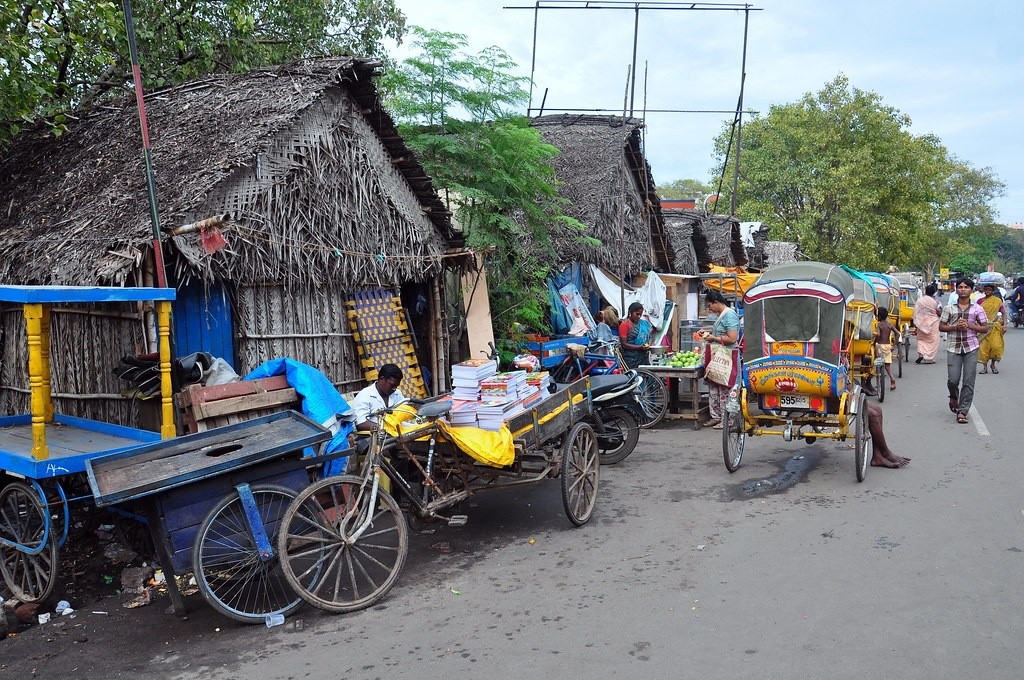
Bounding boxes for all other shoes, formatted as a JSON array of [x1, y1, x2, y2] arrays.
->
[[978, 369, 988, 374], [990, 364, 998, 374], [712, 422, 723, 429], [701, 419, 721, 427]]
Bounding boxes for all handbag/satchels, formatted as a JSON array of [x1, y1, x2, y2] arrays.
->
[[702, 334, 739, 390]]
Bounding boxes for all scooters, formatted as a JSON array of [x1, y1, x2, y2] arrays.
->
[[480, 341, 645, 465]]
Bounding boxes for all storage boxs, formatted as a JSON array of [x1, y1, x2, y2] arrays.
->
[[495, 333, 590, 371]]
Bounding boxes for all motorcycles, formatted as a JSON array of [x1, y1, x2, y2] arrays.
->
[[1007, 297, 1024, 328]]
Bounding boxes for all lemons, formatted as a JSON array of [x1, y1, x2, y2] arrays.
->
[[666, 351, 700, 367]]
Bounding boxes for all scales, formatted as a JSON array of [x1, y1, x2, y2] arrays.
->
[[648, 346, 669, 364]]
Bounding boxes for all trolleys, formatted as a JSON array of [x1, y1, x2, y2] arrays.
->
[[145, 447, 361, 624]]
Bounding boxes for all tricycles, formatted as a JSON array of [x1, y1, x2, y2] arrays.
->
[[844, 264, 919, 403], [277, 379, 600, 614], [515, 334, 668, 429], [722, 261, 872, 483]]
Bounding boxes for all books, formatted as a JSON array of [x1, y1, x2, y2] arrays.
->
[[437, 359, 550, 431]]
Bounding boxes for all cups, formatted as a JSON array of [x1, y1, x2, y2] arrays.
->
[[266, 613, 285, 629]]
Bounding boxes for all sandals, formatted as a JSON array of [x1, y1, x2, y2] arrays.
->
[[957, 410, 968, 423], [949, 397, 959, 413]]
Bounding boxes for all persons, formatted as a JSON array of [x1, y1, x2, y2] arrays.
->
[[350, 363, 420, 510], [696, 291, 741, 429], [836, 277, 1024, 468], [618, 302, 679, 422]]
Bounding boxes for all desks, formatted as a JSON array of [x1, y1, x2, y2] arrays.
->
[[638, 364, 711, 431]]
